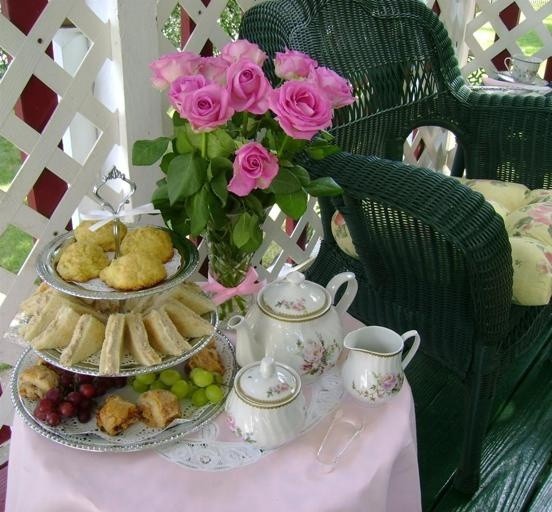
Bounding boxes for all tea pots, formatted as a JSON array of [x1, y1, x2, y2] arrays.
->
[[341, 326, 421, 404], [228, 271, 357, 378]]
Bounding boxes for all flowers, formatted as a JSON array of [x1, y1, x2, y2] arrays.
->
[[133, 40, 356, 316]]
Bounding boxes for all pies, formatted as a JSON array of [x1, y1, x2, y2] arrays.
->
[[17, 366, 59, 402], [96, 394, 141, 437], [136, 388, 182, 428]]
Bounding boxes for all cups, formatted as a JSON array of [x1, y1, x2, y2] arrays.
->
[[505, 55, 543, 85]]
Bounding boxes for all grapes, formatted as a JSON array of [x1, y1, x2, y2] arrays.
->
[[132, 368, 224, 406], [33, 371, 126, 426]]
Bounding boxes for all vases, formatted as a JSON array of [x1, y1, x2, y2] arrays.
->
[[205, 210, 259, 289]]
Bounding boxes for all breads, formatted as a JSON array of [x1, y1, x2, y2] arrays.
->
[[57, 219, 173, 290]]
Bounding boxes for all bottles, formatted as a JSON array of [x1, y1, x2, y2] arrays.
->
[[223, 358, 307, 450]]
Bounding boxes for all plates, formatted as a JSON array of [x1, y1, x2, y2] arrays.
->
[[497, 70, 549, 87]]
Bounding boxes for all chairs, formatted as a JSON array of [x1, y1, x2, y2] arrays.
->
[[239, 0, 552, 494]]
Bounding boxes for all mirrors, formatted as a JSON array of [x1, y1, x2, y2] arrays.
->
[[395, 118, 469, 180]]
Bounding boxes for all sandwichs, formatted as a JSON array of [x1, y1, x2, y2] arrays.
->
[[19, 281, 216, 376]]
